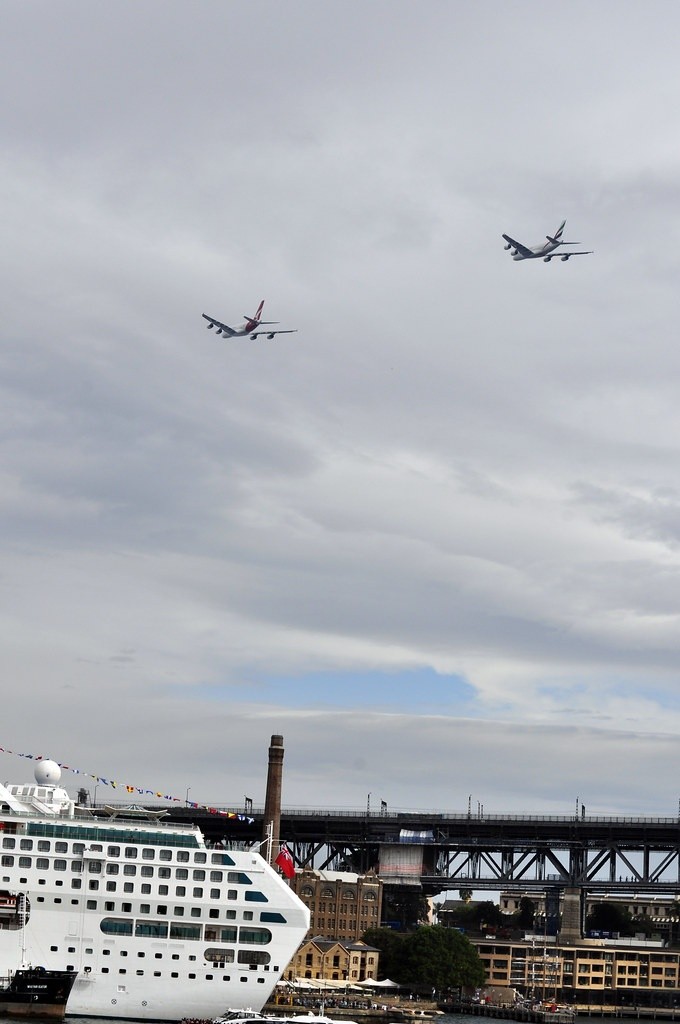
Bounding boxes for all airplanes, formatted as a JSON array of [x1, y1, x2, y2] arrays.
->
[[202, 300, 298, 340], [502, 219, 594, 262]]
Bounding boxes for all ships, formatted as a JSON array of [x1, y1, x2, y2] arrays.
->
[[0, 759, 311, 1024]]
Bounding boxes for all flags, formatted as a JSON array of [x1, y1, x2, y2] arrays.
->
[[0, 748, 255, 824], [275, 844, 297, 880]]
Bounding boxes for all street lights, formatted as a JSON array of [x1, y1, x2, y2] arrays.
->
[[367, 792, 372, 817], [468, 794, 472, 819], [186, 788, 191, 807], [94, 785, 100, 808], [575, 795, 579, 823]]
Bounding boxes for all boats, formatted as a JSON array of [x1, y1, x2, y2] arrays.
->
[[399, 1008, 445, 1020], [181, 992, 357, 1024], [1, 966, 80, 1023]]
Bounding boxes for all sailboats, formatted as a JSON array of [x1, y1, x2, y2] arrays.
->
[[514, 917, 576, 1013]]
